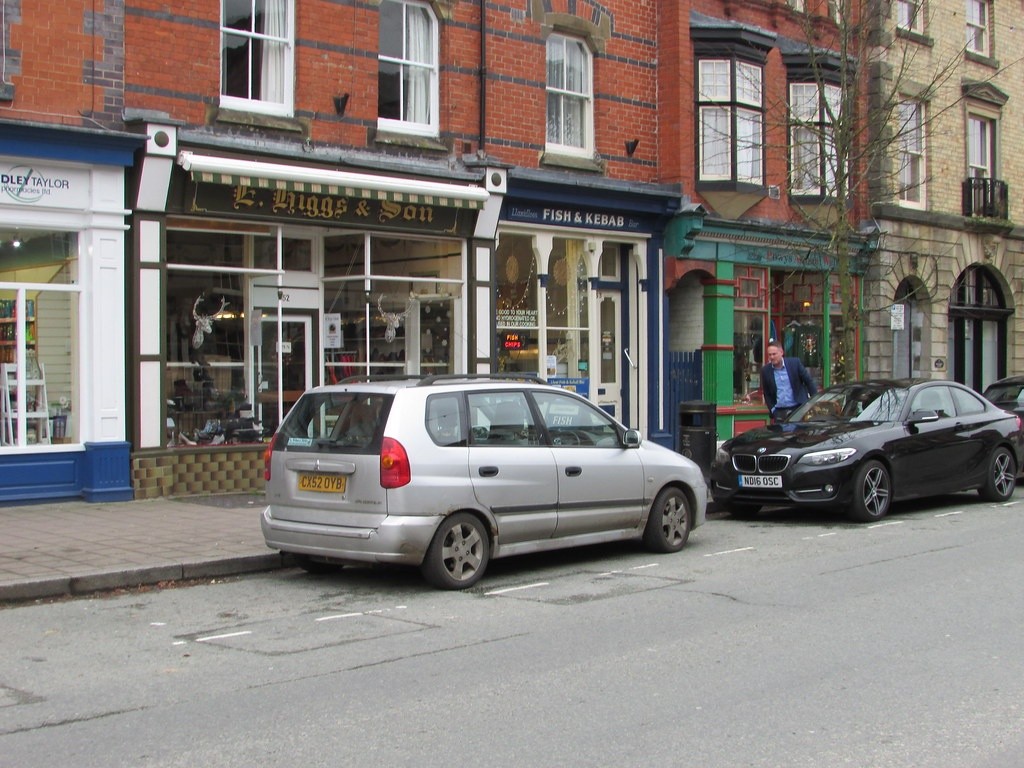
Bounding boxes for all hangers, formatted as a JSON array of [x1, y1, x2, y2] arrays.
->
[[781, 312, 815, 331]]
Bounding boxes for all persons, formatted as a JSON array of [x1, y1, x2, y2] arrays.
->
[[761, 341, 817, 423]]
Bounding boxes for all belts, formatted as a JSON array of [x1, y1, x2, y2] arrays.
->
[[773, 405, 802, 411]]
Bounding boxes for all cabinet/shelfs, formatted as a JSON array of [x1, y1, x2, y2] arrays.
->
[[0, 299, 38, 375], [342, 296, 457, 379], [166, 356, 231, 435]]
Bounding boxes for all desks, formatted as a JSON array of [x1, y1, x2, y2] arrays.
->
[[256, 389, 305, 402]]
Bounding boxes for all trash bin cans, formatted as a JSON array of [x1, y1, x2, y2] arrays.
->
[[677, 399, 718, 490]]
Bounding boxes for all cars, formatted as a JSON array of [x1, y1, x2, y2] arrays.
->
[[0, 387, 71, 446], [260, 373, 708, 590], [983, 375, 1023, 423], [710, 377, 1024, 523]]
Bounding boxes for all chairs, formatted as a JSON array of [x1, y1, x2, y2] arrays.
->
[[486, 399, 528, 447], [921, 391, 946, 416]]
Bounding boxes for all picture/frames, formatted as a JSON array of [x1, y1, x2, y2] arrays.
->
[[410, 271, 440, 296]]
